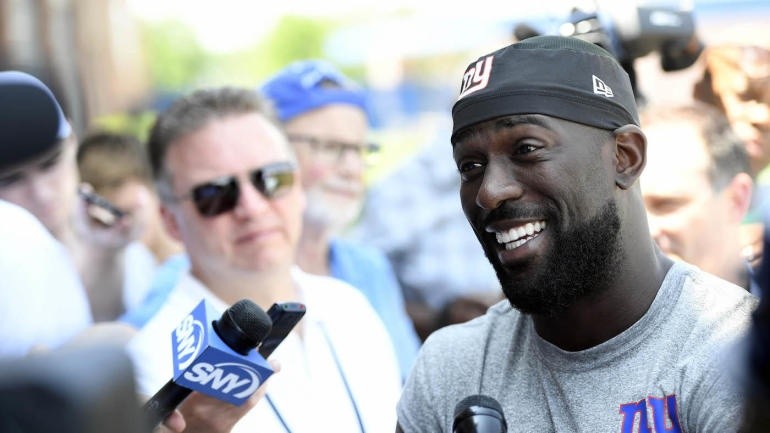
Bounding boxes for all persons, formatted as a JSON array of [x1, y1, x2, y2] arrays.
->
[[0, 71, 188, 363], [363, 132, 506, 330], [394, 34, 761, 433], [129, 87, 402, 433], [265, 63, 421, 391], [639, 22, 770, 296]]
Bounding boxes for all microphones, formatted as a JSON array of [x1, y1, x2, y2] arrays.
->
[[141, 300, 271, 433], [450, 393, 508, 433]]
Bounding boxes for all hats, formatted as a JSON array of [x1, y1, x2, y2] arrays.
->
[[450, 35, 639, 132], [262, 59, 384, 128], [0, 71, 73, 164]]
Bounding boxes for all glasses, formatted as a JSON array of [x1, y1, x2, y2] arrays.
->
[[169, 160, 296, 218], [287, 132, 384, 164]]
